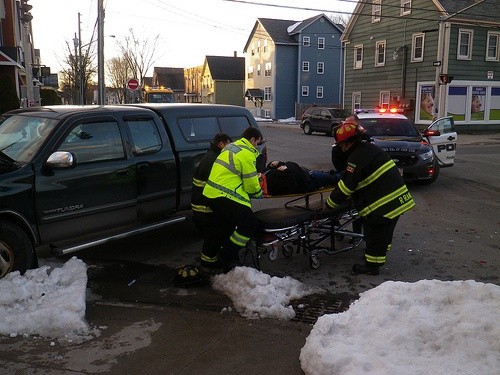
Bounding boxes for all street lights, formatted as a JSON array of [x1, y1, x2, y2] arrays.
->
[[78, 34, 115, 104]]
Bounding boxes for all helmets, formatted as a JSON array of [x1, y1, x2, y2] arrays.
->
[[174, 262, 207, 288], [332, 123, 365, 143]]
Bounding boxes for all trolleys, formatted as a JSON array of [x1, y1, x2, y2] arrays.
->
[[237, 188, 365, 270]]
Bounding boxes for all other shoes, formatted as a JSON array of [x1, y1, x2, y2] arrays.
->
[[352, 259, 381, 275], [199, 257, 224, 268]]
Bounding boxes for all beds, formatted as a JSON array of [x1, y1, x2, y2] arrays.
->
[[243, 191, 365, 270]]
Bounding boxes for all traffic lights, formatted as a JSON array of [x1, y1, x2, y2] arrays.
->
[[439, 73, 455, 83]]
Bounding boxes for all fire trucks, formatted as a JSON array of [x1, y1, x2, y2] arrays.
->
[[143, 85, 175, 104]]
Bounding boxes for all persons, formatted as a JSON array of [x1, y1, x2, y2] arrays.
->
[[257, 160, 346, 196], [200, 127, 265, 268], [323, 114, 417, 275], [190, 132, 240, 263]]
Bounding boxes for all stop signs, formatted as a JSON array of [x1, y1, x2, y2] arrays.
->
[[127, 79, 138, 91]]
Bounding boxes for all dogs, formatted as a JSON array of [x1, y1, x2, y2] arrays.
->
[[471, 95, 482, 113], [422, 93, 434, 116]]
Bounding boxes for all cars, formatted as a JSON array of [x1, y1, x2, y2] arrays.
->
[[331, 108, 458, 187]]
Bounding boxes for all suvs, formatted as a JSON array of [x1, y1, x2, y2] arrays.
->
[[0, 102, 269, 280], [300, 106, 351, 137]]
